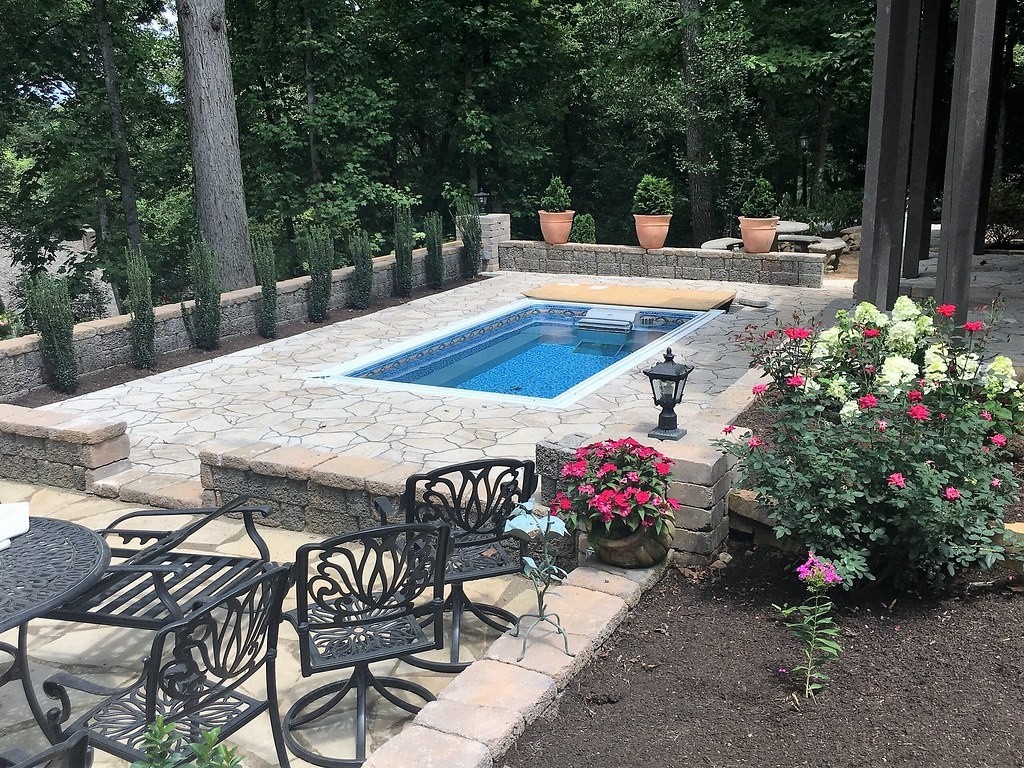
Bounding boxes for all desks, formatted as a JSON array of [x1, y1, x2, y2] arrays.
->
[[0, 517, 111, 768]]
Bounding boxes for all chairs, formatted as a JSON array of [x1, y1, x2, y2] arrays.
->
[[282, 525, 451, 768], [374, 459, 538, 672], [43, 563, 290, 768], [39, 493, 273, 662]]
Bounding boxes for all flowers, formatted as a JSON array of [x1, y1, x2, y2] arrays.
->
[[550, 438, 680, 538]]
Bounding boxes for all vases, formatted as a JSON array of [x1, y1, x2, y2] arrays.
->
[[586, 503, 676, 568]]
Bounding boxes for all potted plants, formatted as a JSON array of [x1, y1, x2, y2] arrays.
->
[[538, 175, 576, 244], [738, 178, 780, 253], [632, 173, 675, 248]]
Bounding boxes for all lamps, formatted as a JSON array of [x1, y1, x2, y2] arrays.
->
[[643, 347, 694, 440], [475, 188, 490, 216]]
